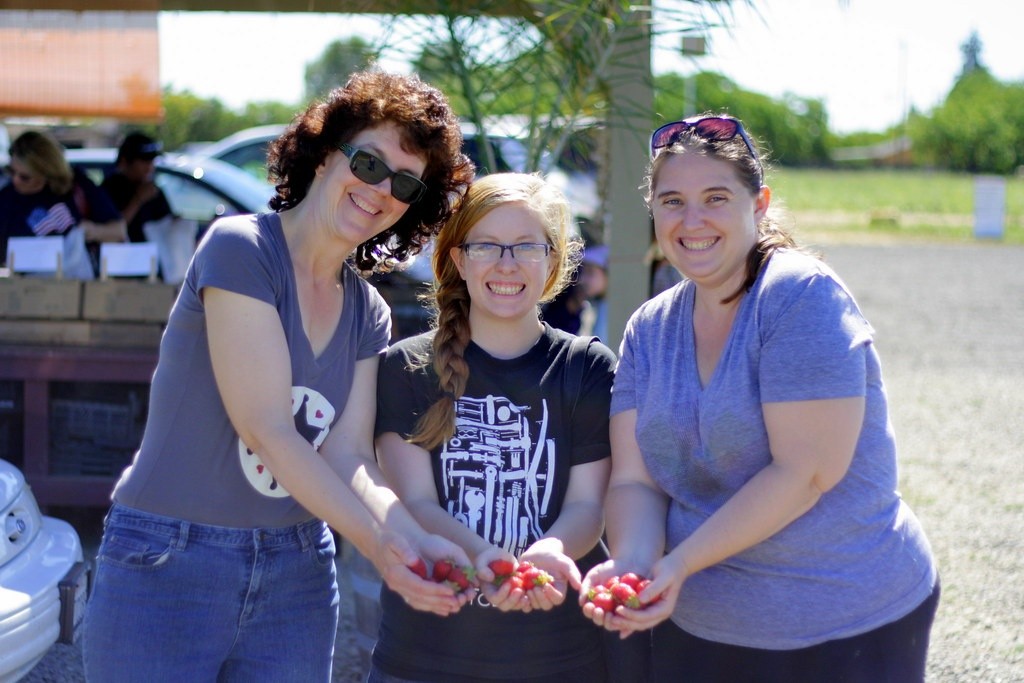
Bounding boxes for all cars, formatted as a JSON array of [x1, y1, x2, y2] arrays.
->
[[1, 150, 442, 348], [486, 109, 606, 244], [0, 458, 94, 683]]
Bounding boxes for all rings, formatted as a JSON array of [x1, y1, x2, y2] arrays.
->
[[491, 601, 499, 609], [404, 596, 414, 608]]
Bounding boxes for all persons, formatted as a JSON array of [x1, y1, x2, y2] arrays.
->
[[0, 130, 129, 470], [576, 109, 941, 682], [79, 69, 475, 683], [99, 128, 186, 420], [367, 169, 619, 683]]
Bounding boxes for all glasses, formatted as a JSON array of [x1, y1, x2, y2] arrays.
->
[[5, 165, 45, 182], [458, 239, 556, 262], [339, 146, 428, 206], [649, 117, 760, 167]]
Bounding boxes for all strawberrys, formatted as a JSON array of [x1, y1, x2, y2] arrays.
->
[[411, 558, 552, 596], [586, 571, 658, 614]]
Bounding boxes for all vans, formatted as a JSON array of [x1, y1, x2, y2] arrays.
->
[[198, 122, 570, 267]]
